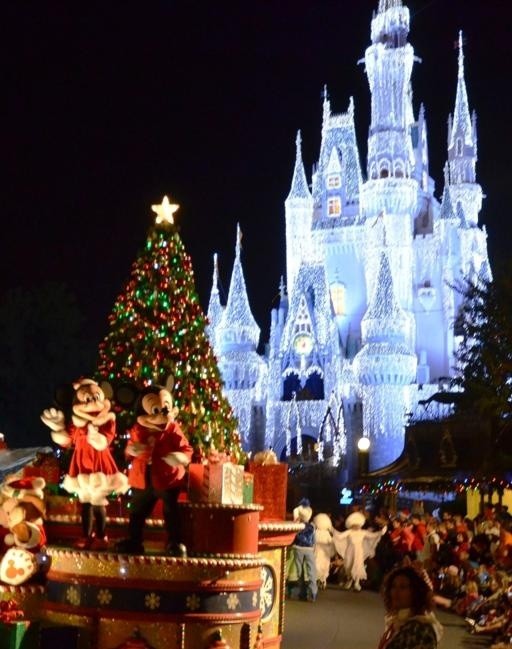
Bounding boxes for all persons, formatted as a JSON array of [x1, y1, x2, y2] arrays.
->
[[327, 498, 512, 649], [285, 497, 387, 602]]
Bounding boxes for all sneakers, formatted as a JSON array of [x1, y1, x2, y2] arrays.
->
[[353, 584, 362, 591], [343, 581, 353, 590]]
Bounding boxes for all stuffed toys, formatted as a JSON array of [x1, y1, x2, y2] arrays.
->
[[40, 374, 131, 551], [113, 374, 194, 556]]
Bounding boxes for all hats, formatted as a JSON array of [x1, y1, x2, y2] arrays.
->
[[293, 505, 313, 524], [313, 513, 332, 529], [346, 512, 367, 528]]
